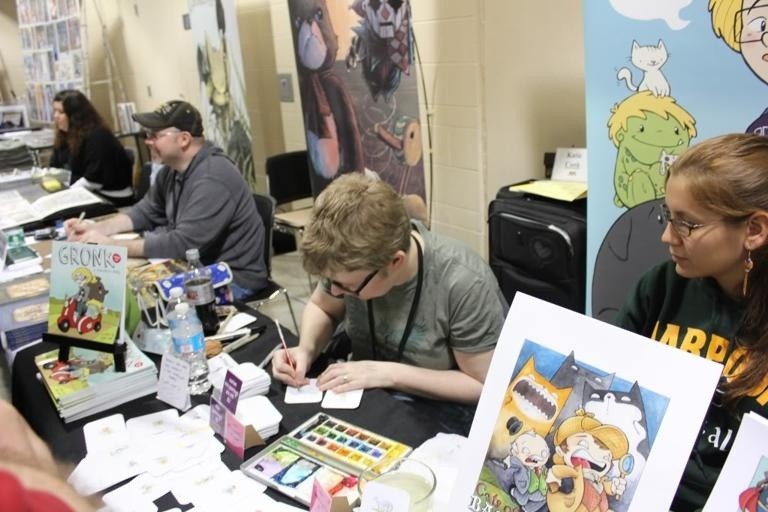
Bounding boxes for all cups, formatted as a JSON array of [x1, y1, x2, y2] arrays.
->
[[355, 457, 439, 512]]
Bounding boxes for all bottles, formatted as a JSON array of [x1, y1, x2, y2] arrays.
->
[[165, 287, 216, 397], [181, 246, 220, 336]]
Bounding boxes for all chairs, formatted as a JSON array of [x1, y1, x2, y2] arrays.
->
[[265, 150, 315, 253], [124, 145, 138, 183], [246, 193, 301, 339]]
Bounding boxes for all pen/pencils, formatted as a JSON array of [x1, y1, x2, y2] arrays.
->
[[258, 343, 284, 369], [204, 324, 268, 353], [66, 212, 86, 243]]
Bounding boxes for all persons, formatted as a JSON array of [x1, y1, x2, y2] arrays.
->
[[271, 170, 508, 438], [0, 400, 94, 512], [47, 90, 136, 207], [614, 133, 768, 512], [62, 101, 268, 300]]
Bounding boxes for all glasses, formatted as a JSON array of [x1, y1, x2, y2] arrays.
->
[[141, 127, 177, 139], [660, 203, 724, 237], [331, 269, 377, 296]]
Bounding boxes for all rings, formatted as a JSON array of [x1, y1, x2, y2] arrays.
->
[[344, 374, 348, 382]]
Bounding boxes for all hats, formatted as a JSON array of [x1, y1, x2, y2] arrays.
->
[[133, 101, 203, 135]]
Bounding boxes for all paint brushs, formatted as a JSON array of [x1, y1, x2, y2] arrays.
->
[[274, 319, 300, 392]]
